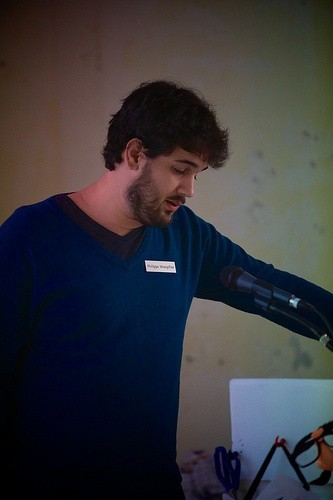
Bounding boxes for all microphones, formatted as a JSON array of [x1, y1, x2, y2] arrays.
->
[[220, 264, 313, 323]]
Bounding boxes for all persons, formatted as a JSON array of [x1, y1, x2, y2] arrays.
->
[[0, 79, 332, 499]]
